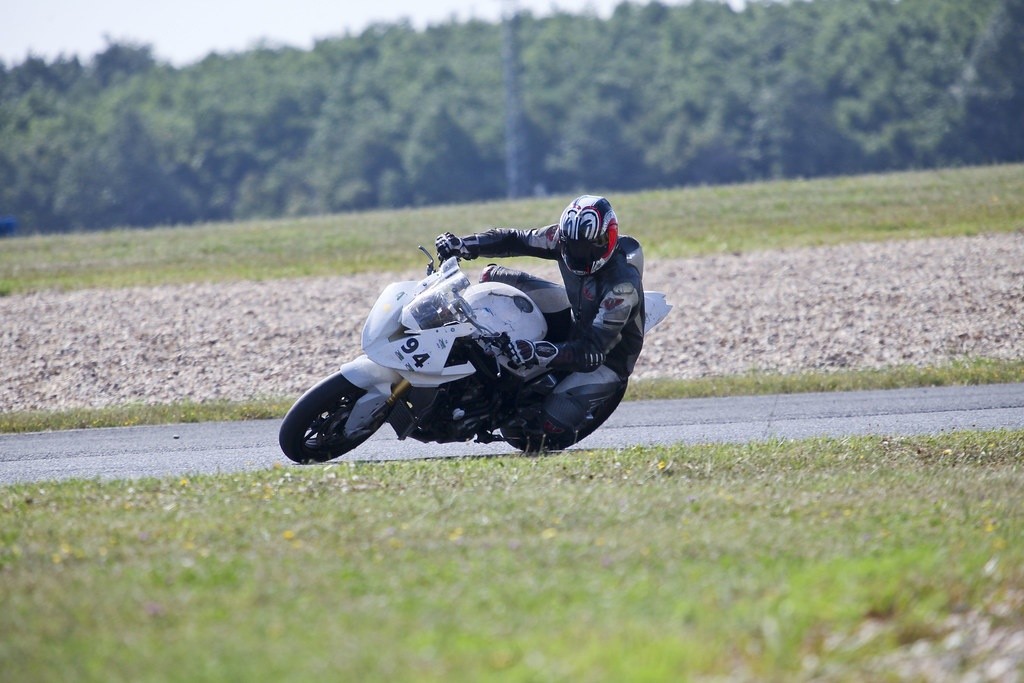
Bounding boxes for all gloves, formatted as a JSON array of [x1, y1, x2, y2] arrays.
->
[[504, 339, 557, 369], [434, 232, 479, 261]]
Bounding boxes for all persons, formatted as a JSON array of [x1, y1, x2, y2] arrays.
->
[[436, 195, 646, 439]]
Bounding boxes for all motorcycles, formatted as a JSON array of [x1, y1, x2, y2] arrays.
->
[[277, 230, 673, 465]]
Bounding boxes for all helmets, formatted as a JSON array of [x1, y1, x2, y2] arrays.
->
[[558, 195, 621, 277]]
